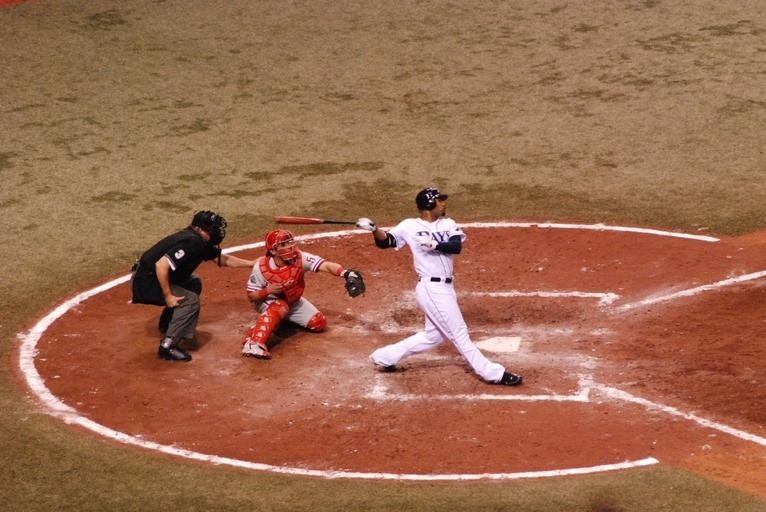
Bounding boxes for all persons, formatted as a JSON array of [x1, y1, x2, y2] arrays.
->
[[131, 210, 266, 363], [352, 187, 526, 388], [241, 230, 365, 358]]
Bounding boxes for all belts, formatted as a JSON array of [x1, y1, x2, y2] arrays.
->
[[430, 277, 453, 284]]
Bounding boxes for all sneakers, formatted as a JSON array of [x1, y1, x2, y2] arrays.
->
[[242, 337, 272, 358], [494, 372, 521, 385], [370, 355, 394, 371]]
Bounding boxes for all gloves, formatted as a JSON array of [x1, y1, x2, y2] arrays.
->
[[340, 269, 359, 278], [356, 218, 376, 232], [418, 237, 439, 252]]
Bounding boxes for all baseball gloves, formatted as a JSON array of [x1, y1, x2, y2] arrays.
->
[[345, 269, 365, 296]]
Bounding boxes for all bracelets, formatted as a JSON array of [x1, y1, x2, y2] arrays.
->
[[259, 286, 268, 300]]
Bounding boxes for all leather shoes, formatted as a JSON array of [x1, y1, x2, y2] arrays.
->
[[159, 345, 191, 361]]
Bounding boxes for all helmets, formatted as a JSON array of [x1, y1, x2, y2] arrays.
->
[[265, 229, 298, 261], [415, 187, 448, 210], [192, 210, 226, 245]]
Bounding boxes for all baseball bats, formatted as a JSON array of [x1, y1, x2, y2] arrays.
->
[[275, 216, 375, 227]]
